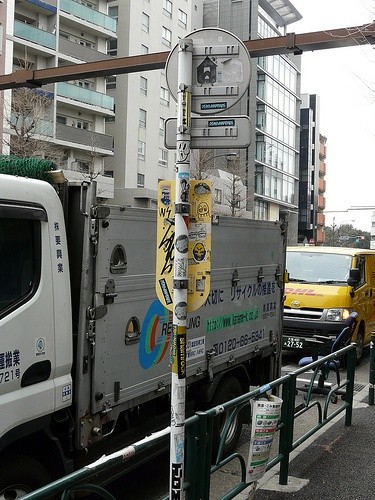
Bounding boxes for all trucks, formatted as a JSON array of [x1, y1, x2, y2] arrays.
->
[[0, 173, 288, 500]]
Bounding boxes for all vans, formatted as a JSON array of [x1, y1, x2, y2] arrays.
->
[[282, 246, 375, 367]]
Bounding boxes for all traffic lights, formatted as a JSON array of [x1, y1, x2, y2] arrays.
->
[[355, 235, 364, 240]]
